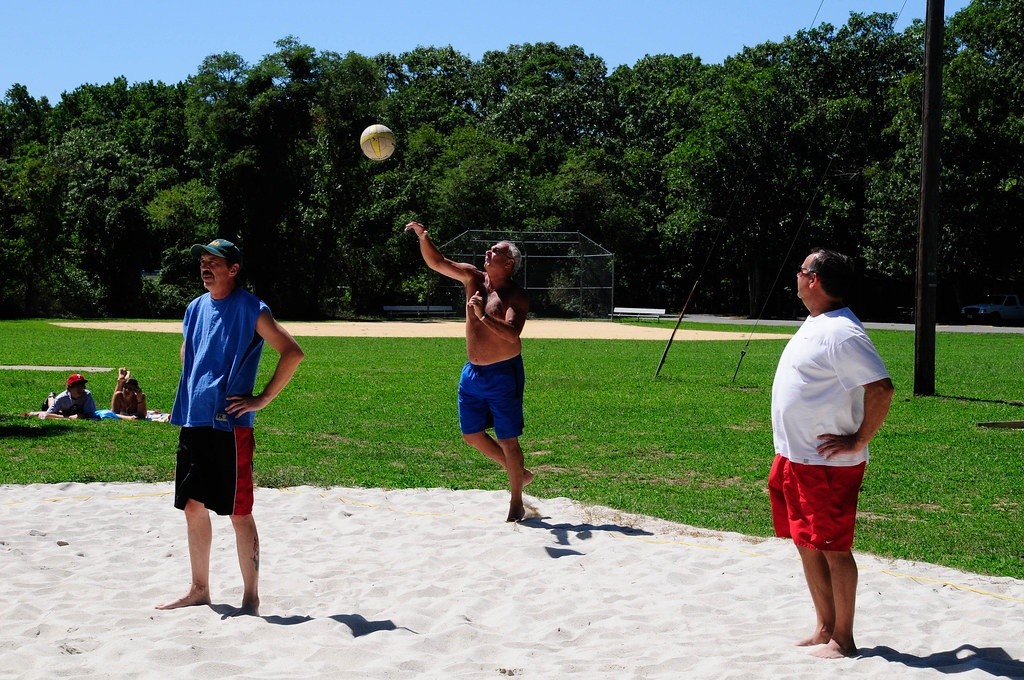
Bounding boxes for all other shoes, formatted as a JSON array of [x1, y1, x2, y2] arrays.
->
[[42, 392, 54, 411]]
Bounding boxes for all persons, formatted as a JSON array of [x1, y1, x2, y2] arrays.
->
[[767, 251, 894, 660], [42, 374, 97, 419], [111, 367, 147, 421], [154, 238, 304, 616], [405, 221, 534, 522]]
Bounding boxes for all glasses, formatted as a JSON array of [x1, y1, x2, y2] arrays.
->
[[797, 265, 818, 276]]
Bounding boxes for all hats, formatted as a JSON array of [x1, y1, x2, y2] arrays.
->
[[191, 239, 239, 260], [123, 379, 138, 390], [67, 374, 87, 387]]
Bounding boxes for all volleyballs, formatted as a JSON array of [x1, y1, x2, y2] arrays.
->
[[360, 124, 396, 161]]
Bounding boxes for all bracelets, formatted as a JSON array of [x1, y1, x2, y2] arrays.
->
[[137, 402, 142, 404], [480, 313, 488, 321]]
